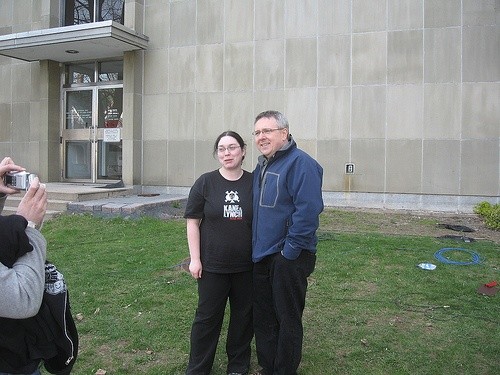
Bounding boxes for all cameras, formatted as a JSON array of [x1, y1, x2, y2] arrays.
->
[[4, 171, 36, 190]]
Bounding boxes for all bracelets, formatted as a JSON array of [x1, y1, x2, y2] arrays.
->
[[26, 221, 42, 232]]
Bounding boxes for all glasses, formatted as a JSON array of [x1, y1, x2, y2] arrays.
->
[[218, 145, 241, 152], [252, 127, 283, 136]]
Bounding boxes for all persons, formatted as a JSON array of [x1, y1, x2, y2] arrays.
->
[[0, 156, 48, 375], [183, 131, 255, 375], [253, 110, 324, 375]]
